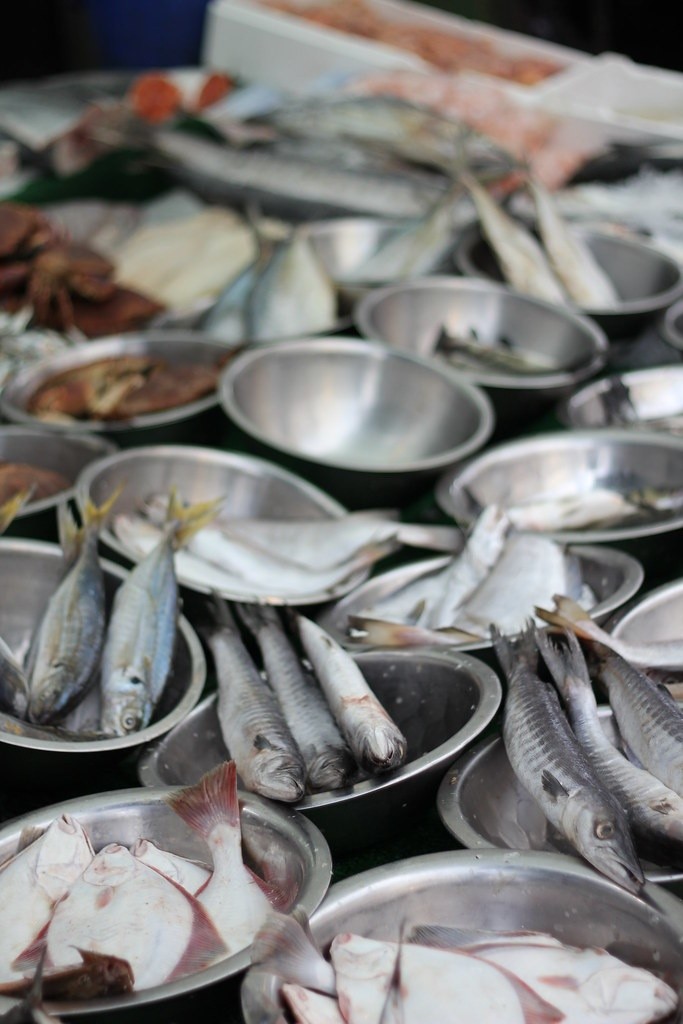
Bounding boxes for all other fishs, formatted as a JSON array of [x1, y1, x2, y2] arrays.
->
[[0, 98, 683, 1024]]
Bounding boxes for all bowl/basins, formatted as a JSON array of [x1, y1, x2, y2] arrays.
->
[[0, 211, 683, 1024]]
